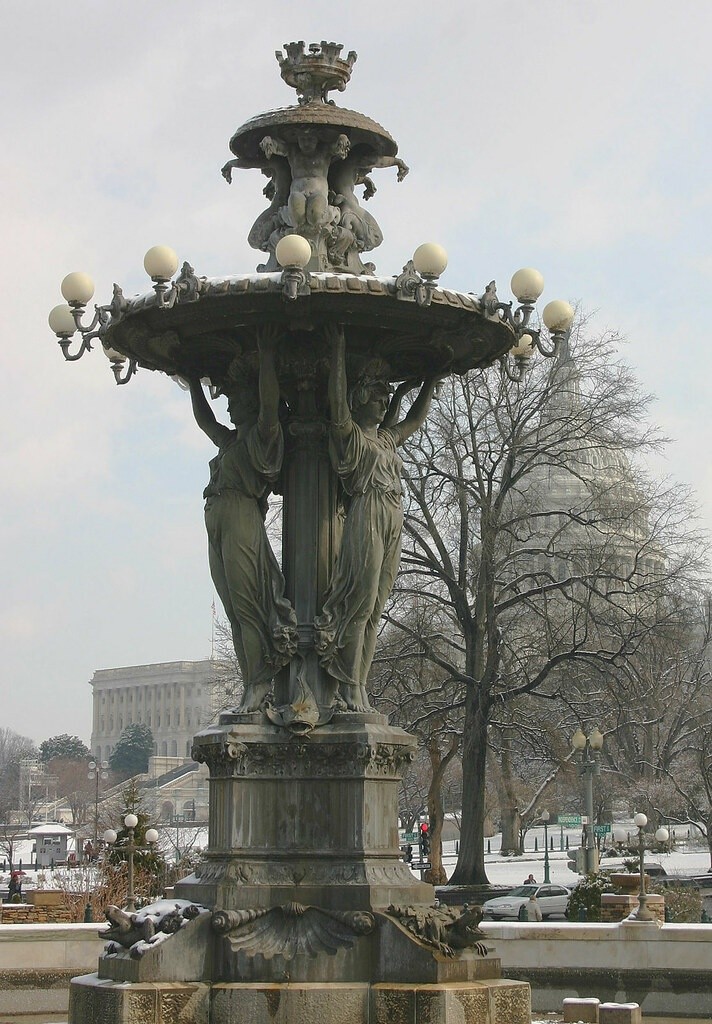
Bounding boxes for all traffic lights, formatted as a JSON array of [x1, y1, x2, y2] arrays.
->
[[404, 846, 412, 863], [566, 848, 586, 873], [420, 823, 430, 855]]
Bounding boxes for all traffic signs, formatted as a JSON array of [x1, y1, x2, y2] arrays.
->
[[411, 863, 432, 870], [591, 825, 611, 837], [557, 815, 581, 828]]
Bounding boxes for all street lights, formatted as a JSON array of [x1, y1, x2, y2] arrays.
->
[[87, 758, 109, 851], [542, 808, 552, 883], [103, 815, 160, 912], [571, 726, 603, 881], [613, 812, 670, 921]]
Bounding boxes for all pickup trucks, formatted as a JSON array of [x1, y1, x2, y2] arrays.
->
[[596, 862, 712, 896]]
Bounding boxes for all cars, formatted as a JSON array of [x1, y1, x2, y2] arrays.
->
[[480, 882, 584, 920]]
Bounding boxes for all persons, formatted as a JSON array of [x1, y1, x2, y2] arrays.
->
[[321, 323, 455, 706], [5, 875, 22, 901], [210, 132, 412, 252], [524, 894, 543, 922], [523, 874, 536, 885], [148, 325, 303, 715]]
